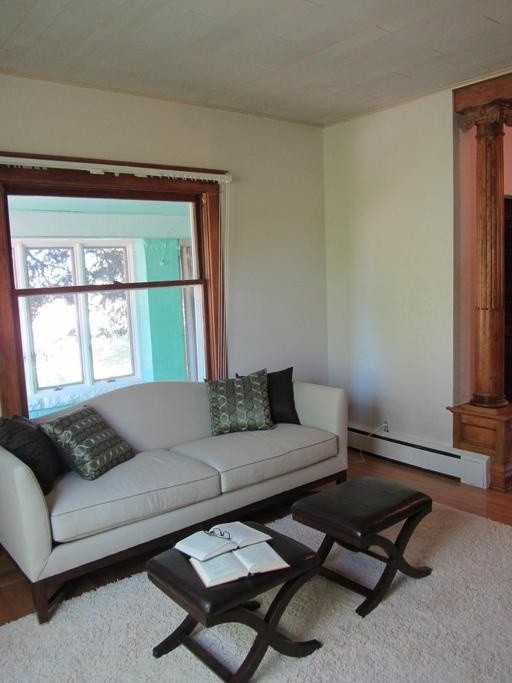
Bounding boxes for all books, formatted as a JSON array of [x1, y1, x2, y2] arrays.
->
[[187, 541, 292, 588], [173, 520, 275, 560]]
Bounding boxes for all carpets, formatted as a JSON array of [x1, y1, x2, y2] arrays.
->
[[0, 501, 511, 683]]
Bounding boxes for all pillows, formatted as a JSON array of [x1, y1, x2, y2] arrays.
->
[[203, 365, 302, 437], [1, 403, 138, 496]]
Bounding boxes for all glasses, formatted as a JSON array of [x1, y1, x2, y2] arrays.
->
[[203, 528, 230, 540]]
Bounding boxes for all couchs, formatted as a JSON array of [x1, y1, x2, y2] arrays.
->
[[1, 379, 349, 625]]
